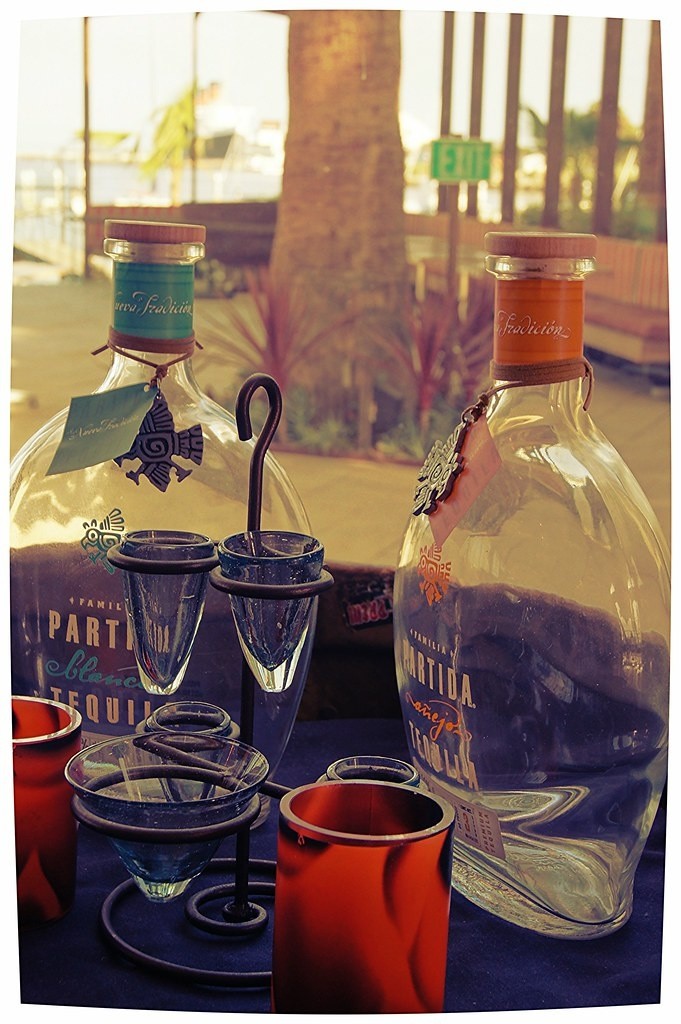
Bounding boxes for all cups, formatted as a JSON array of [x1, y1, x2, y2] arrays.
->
[[269, 778, 458, 1014], [11, 695, 84, 934]]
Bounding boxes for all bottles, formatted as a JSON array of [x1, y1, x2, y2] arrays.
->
[[11, 220, 321, 836], [390, 231, 670, 943]]
